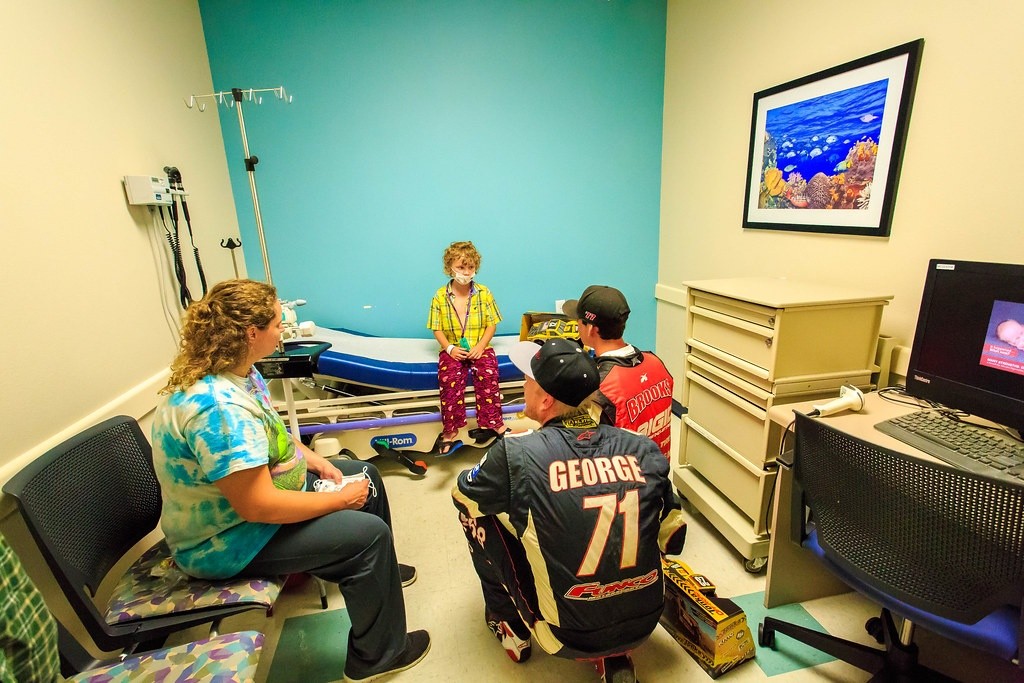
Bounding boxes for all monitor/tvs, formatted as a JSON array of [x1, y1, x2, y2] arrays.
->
[[906, 259, 1024, 444]]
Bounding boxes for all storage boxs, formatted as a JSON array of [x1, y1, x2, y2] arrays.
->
[[660, 555, 756, 680]]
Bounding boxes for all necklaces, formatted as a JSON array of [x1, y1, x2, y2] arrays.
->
[[445, 283, 473, 353]]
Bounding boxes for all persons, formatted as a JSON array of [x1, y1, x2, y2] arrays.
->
[[0, 531, 61, 683], [151, 279, 432, 683], [428, 241, 516, 461], [453, 337, 674, 683], [562, 284, 673, 472], [996, 318, 1024, 350]]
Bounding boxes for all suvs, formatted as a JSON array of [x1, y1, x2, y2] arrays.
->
[[527, 319, 580, 346]]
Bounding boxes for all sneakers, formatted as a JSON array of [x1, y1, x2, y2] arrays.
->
[[398, 564, 416, 587], [597, 653, 639, 683], [343, 630, 431, 683], [485, 605, 532, 662]]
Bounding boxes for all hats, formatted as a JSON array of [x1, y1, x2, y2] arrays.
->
[[562, 285, 631, 326], [508, 338, 599, 408]]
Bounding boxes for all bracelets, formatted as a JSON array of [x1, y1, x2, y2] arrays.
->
[[447, 345, 455, 355]]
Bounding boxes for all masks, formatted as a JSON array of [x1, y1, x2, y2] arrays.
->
[[450, 266, 474, 285], [313, 466, 377, 497]]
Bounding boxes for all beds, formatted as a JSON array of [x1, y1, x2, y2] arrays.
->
[[265, 307, 542, 474]]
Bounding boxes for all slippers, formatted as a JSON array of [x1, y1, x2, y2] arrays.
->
[[434, 439, 463, 457]]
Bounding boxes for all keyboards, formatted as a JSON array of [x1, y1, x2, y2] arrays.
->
[[873, 409, 1024, 485]]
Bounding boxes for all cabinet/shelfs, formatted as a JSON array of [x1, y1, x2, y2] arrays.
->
[[671, 286, 895, 571]]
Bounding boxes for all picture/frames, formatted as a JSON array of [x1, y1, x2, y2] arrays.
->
[[741, 37, 926, 238]]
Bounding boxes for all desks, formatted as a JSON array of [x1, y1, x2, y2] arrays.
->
[[762, 386, 1024, 646]]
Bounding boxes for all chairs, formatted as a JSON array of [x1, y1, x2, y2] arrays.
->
[[757, 408, 1024, 683]]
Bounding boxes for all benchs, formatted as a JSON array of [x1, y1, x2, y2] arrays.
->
[[0, 415, 328, 683]]
